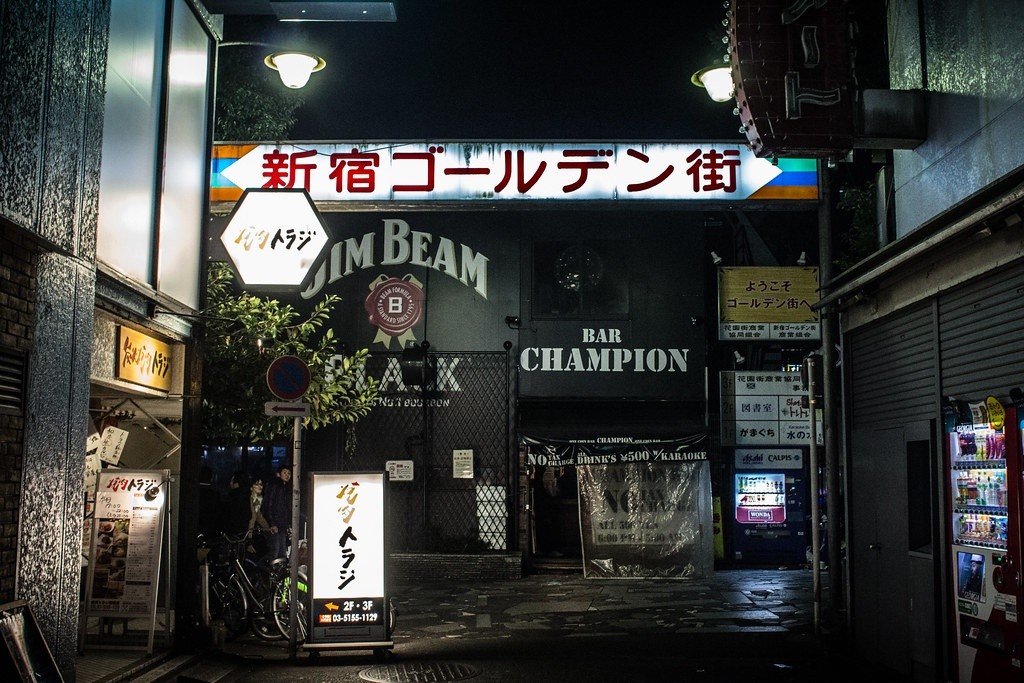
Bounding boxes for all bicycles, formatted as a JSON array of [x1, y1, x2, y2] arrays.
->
[[194, 527, 396, 650]]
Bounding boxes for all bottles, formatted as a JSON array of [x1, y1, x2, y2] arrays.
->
[[957, 429, 1006, 463], [779, 481, 783, 491], [757, 495, 761, 505], [761, 494, 766, 504], [774, 481, 779, 490], [738, 476, 766, 492], [766, 481, 770, 491], [956, 470, 1007, 507], [770, 481, 773, 491], [959, 514, 1007, 542], [775, 494, 783, 504]]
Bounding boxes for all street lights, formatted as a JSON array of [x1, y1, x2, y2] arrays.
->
[[693, 64, 842, 587]]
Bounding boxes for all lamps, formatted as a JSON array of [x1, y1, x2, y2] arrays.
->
[[692, 53, 735, 102], [218, 40, 326, 88]]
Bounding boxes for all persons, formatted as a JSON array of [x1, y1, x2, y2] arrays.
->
[[197, 465, 292, 579]]
